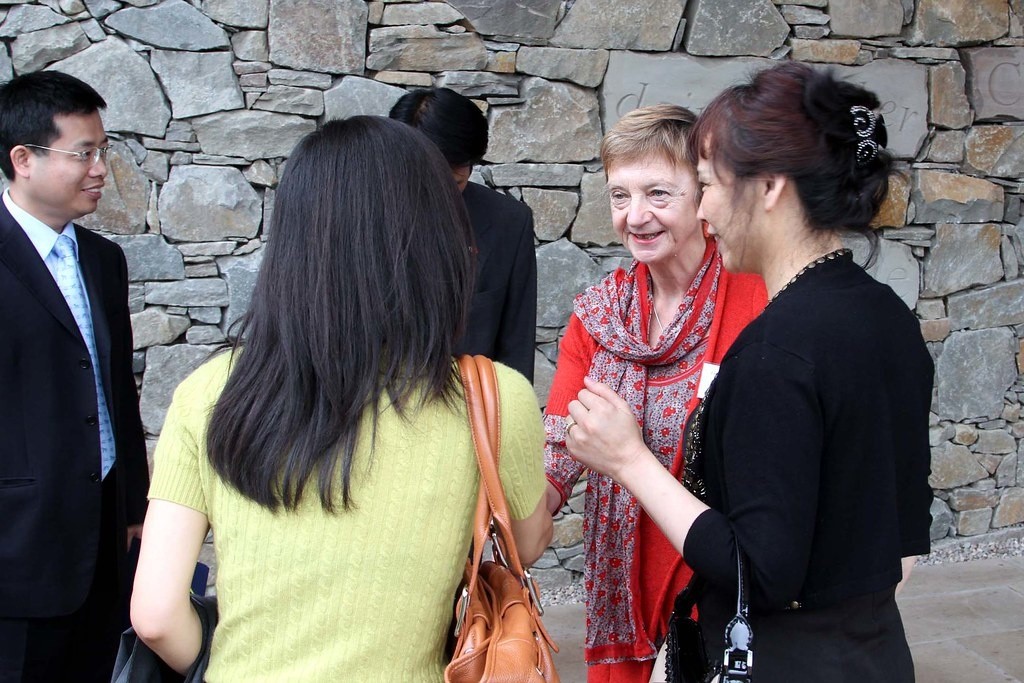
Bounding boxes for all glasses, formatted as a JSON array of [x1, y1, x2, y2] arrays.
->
[[21, 142, 114, 167]]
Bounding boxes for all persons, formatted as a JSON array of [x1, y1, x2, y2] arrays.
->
[[383, 84, 540, 390], [532, 101, 771, 683], [126, 114, 556, 683], [557, 62, 936, 683], [0, 69, 152, 683]]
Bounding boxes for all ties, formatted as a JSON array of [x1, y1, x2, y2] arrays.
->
[[54, 236, 116, 482]]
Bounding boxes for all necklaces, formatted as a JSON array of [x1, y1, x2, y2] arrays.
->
[[650, 293, 666, 336], [761, 244, 852, 314]]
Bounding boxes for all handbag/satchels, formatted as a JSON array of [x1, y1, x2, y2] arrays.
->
[[650, 522, 753, 683], [448, 349, 565, 683]]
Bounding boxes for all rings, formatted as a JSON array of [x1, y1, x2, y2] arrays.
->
[[562, 419, 578, 436]]
